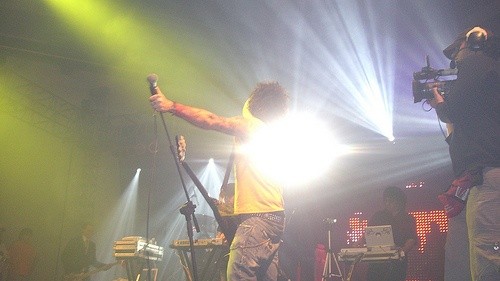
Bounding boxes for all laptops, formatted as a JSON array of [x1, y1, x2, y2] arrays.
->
[[365, 225, 398, 247]]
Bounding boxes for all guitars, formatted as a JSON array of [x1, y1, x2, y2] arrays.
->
[[175, 134, 238, 244], [62, 260, 122, 281]]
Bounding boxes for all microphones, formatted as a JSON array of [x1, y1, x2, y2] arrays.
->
[[147, 73, 158, 97]]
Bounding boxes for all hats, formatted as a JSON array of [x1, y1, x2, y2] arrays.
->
[[442, 25, 495, 60]]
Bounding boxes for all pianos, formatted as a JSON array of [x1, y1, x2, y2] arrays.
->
[[169, 239, 230, 281]]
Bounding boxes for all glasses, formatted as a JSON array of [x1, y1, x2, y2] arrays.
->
[[452, 46, 470, 57]]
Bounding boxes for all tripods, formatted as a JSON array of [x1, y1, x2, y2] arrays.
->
[[321, 222, 344, 281]]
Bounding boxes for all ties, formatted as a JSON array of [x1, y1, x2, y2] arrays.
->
[[85, 240, 88, 255]]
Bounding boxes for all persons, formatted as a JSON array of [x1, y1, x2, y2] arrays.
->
[[354, 186, 417, 281], [428, 24, 500, 281], [61, 222, 112, 281], [148, 82, 288, 281], [9, 228, 42, 281], [219, 182, 236, 208]]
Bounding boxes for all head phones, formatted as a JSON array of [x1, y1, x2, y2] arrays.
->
[[469, 25, 486, 50]]
